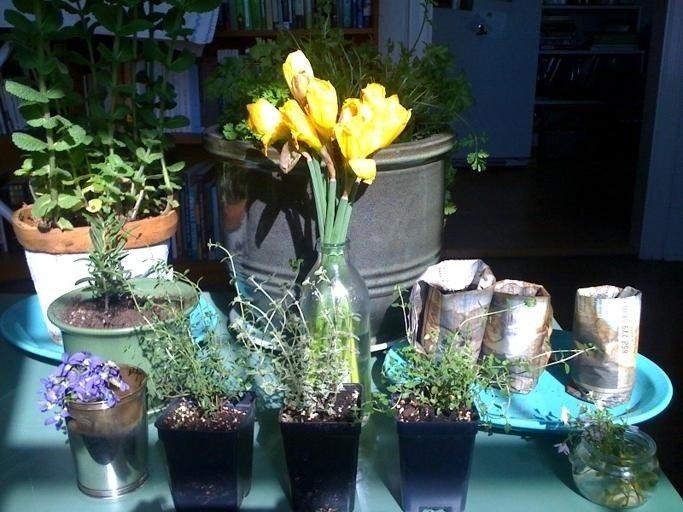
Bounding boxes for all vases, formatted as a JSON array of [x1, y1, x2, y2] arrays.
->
[[66, 362, 150, 498], [284, 239, 372, 400]]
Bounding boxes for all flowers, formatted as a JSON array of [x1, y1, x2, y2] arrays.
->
[[38, 352, 128, 431], [241, 51, 418, 245]]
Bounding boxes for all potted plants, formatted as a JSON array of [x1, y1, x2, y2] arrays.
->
[[533, 385, 660, 512], [42, 206, 203, 393], [369, 278, 602, 512], [203, 11, 487, 346], [117, 256, 266, 512], [2, 1, 219, 347], [226, 251, 369, 512]]
